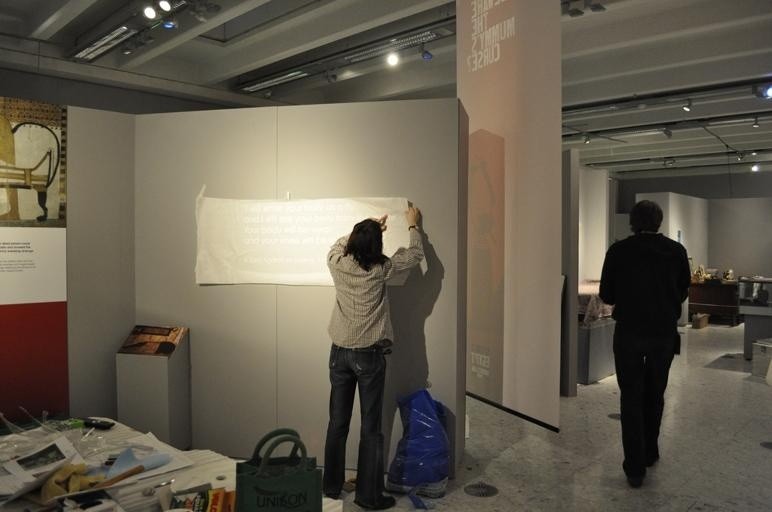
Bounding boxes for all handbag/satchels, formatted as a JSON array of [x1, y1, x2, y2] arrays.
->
[[234, 428, 323, 512]]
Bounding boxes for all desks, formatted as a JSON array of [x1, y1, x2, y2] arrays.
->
[[689, 279, 740, 327], [0, 411, 345, 511]]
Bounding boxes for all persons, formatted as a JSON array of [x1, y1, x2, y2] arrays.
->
[[598, 199, 691, 488], [321, 204, 427, 511]]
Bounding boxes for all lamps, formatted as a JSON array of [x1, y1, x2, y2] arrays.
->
[[419, 48, 433, 60], [752, 122, 759, 127], [682, 103, 691, 112], [761, 86, 772, 98]]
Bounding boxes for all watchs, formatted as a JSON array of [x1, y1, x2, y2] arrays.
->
[[408, 225, 419, 231]]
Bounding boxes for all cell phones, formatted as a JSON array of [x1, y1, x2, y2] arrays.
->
[[81, 417, 116, 429]]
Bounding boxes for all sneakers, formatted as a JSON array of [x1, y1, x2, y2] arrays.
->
[[621, 447, 659, 488], [354, 494, 396, 509], [324, 487, 340, 500]]
